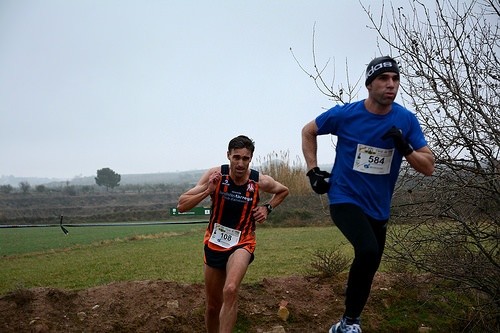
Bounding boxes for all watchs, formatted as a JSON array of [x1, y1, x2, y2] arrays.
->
[[264, 204, 272, 214]]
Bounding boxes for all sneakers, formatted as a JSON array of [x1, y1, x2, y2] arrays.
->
[[328, 319, 363, 333]]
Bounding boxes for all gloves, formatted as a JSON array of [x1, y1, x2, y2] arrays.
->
[[383, 126, 415, 157], [304, 167, 333, 195]]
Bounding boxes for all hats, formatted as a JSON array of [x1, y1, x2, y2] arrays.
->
[[365, 55, 401, 89]]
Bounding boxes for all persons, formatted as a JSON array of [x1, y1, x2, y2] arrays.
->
[[302, 56, 434, 333], [177, 135, 289, 333]]
